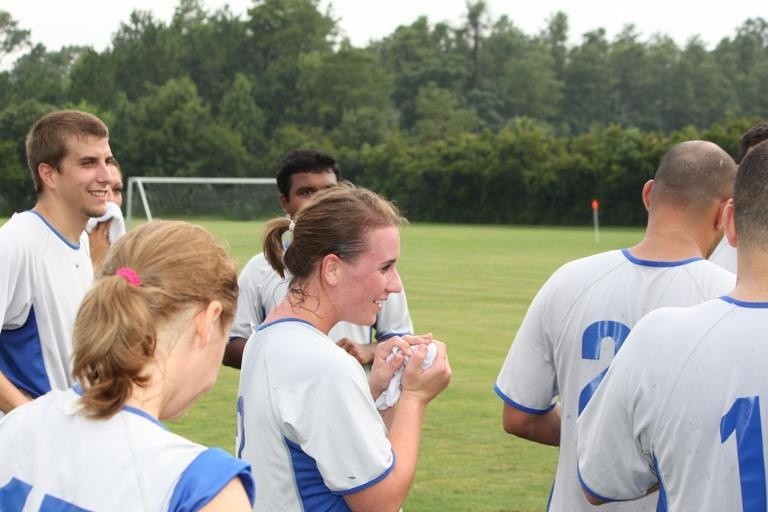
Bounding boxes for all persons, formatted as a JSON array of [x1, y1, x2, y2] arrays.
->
[[0, 218, 258, 512], [234, 182, 453, 512], [491, 139, 738, 512], [0, 110, 112, 417], [226, 148, 415, 379], [84, 155, 123, 276], [707, 122, 768, 274], [574, 142, 768, 512]]
